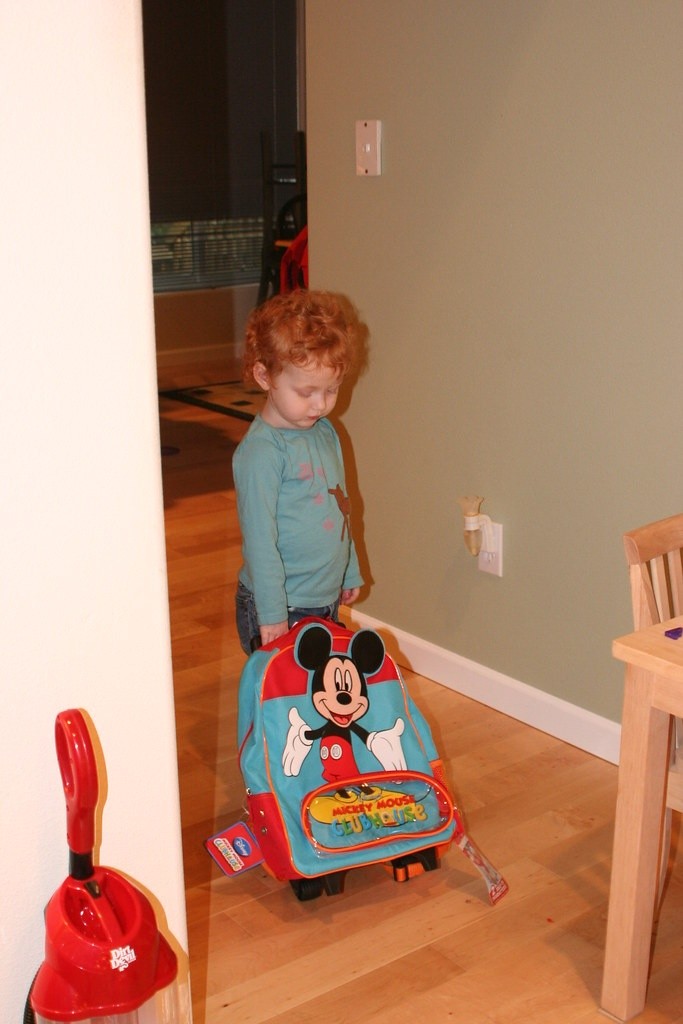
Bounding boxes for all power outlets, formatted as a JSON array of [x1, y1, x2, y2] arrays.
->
[[478, 523, 504, 577]]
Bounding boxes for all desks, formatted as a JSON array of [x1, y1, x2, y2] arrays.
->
[[600, 615, 683, 1024], [256, 241, 292, 310]]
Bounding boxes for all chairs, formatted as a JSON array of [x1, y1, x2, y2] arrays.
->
[[622, 512, 683, 632], [278, 193, 307, 240]]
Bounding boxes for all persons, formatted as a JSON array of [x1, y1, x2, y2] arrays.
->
[[236, 290, 364, 659]]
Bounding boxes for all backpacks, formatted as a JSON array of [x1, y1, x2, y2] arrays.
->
[[236, 614, 468, 900]]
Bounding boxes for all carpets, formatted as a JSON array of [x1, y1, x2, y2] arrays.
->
[[158, 380, 267, 424]]
